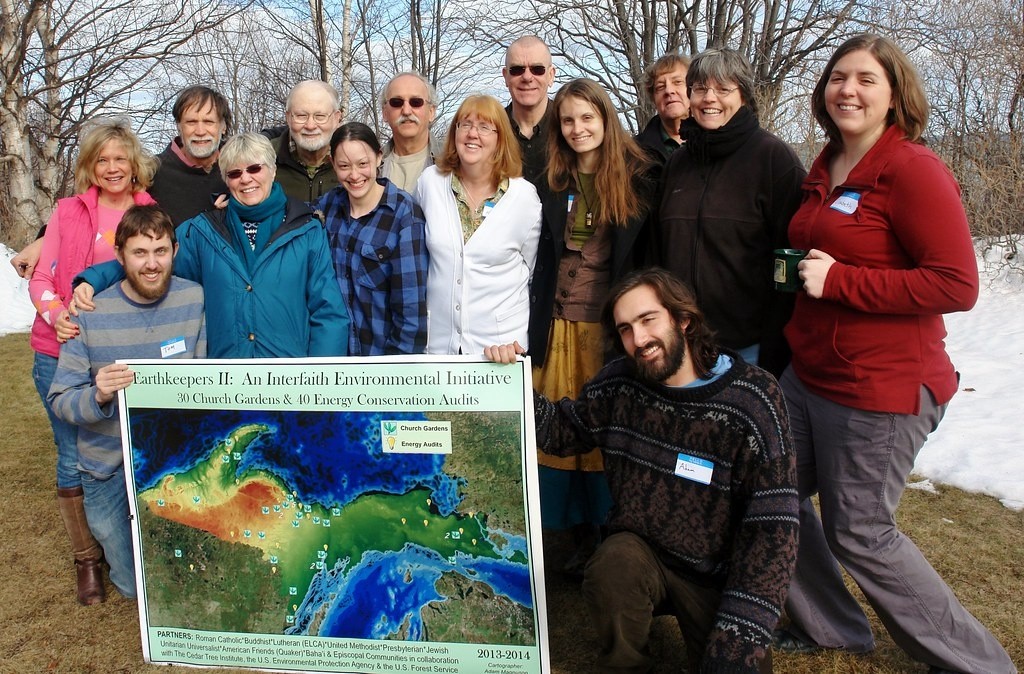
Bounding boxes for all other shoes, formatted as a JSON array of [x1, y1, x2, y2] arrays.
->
[[772, 630, 819, 652]]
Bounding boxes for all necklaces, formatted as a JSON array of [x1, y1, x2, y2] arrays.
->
[[575, 157, 594, 228], [459, 178, 489, 213]]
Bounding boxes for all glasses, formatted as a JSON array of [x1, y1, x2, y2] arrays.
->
[[456, 120, 499, 135], [292, 110, 334, 124], [385, 98, 431, 107], [225, 164, 266, 178], [690, 84, 740, 96], [507, 65, 551, 76]]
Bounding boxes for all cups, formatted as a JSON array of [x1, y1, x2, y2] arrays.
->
[[774, 249, 807, 292]]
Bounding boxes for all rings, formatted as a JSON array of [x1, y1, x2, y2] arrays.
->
[[70, 299, 75, 303]]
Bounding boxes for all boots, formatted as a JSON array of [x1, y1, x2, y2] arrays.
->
[[57, 484, 104, 605]]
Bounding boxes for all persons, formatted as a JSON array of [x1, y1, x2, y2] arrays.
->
[[501, 36, 553, 184], [28, 117, 157, 606], [68, 132, 351, 358], [483, 267, 799, 674], [377, 72, 444, 196], [416, 95, 541, 356], [210, 122, 429, 356], [10, 86, 231, 280], [653, 47, 808, 376], [530, 80, 660, 597], [634, 55, 690, 168], [47, 205, 206, 598], [767, 34, 1017, 674], [260, 80, 341, 204]]
